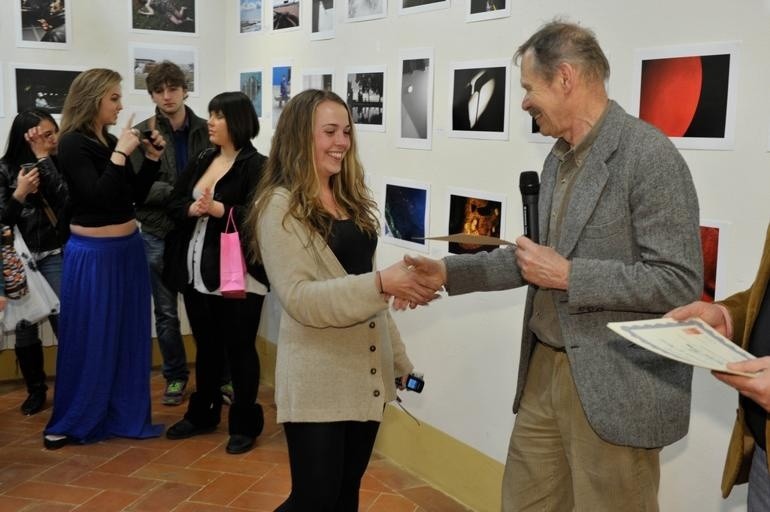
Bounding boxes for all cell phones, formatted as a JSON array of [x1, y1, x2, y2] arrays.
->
[[143, 130, 163, 152]]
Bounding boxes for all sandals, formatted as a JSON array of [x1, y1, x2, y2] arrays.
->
[[44, 434, 67, 450]]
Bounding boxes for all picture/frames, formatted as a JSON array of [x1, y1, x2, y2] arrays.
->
[[127, 42, 199, 98], [447, 58, 513, 141], [310, 0, 337, 42], [270, 63, 294, 134], [378, 181, 431, 255], [343, 68, 387, 133], [525, 42, 610, 145], [345, 0, 389, 23], [127, 0, 201, 38], [238, 1, 264, 35], [238, 70, 264, 118], [10, 62, 82, 120], [300, 72, 336, 92], [463, 0, 512, 25], [444, 191, 507, 256], [396, 51, 435, 150], [270, 1, 302, 33], [701, 219, 730, 302], [628, 38, 743, 152], [14, 0, 72, 51], [398, 1, 452, 16]]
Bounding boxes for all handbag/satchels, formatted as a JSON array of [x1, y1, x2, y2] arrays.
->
[[220, 207, 246, 300], [0, 225, 29, 300]]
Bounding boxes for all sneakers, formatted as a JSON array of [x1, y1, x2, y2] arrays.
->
[[221, 385, 234, 406], [160, 380, 188, 405]]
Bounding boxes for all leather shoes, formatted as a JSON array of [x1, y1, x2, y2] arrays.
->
[[226, 433, 252, 454], [167, 417, 216, 439], [20, 392, 47, 416]]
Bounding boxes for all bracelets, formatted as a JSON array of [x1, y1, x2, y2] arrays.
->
[[376, 270, 384, 292], [113, 148, 129, 158]]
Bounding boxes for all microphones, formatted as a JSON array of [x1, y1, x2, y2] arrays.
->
[[519, 169, 541, 247]]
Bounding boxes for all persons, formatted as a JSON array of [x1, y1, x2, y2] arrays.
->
[[166, 92, 270, 454], [126, 60, 235, 408], [393, 22, 703, 511], [662, 222, 770, 512], [0, 106, 68, 415], [246, 89, 445, 512], [43, 68, 165, 450]]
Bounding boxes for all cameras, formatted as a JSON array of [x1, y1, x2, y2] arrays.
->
[[395, 375, 425, 394]]
